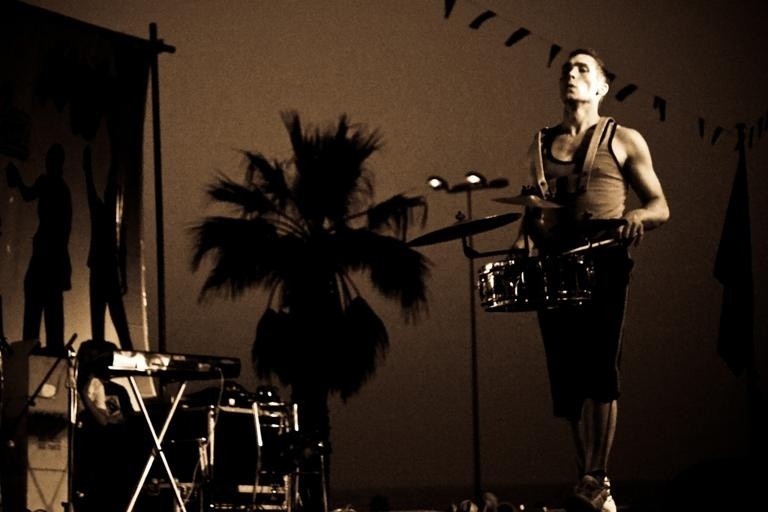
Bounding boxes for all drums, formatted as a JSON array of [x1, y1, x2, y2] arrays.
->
[[477, 252, 594, 312]]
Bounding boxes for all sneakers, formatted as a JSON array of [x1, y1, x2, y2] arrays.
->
[[564, 474, 617, 512]]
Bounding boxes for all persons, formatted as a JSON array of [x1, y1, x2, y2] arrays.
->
[[505, 48, 670, 512], [77, 340, 135, 428]]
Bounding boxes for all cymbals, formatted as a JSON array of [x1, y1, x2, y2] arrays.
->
[[404, 211, 522, 247], [490, 195, 561, 208]]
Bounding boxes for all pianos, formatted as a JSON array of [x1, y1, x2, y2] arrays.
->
[[76, 339, 241, 380]]
